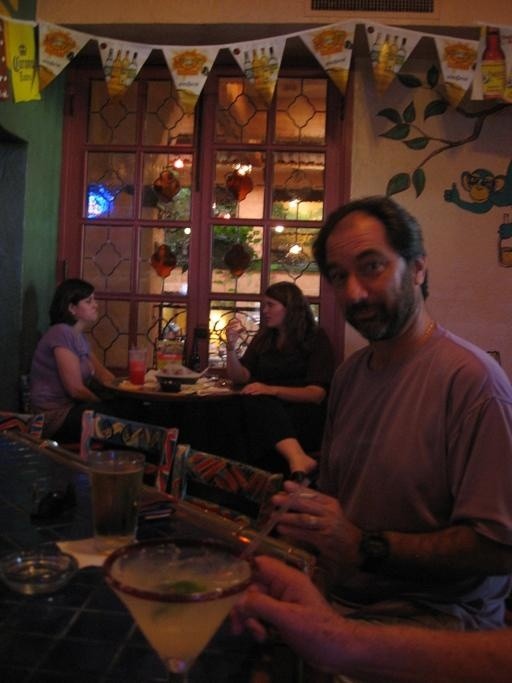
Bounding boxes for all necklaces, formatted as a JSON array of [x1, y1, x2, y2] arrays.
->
[[412, 320, 436, 353]]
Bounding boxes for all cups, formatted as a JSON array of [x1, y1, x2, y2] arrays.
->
[[86, 447, 146, 538], [101, 533, 259, 679], [126, 348, 147, 385]]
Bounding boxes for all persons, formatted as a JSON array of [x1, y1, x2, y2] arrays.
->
[[226, 280, 337, 483], [30, 278, 142, 443], [229, 553, 512, 683], [272, 194, 511, 628]]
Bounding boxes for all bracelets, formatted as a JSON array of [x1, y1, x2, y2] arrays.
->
[[226, 343, 234, 352]]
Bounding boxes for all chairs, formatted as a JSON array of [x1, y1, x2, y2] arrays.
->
[[1, 411, 47, 439], [168, 446, 282, 524], [80, 410, 179, 495], [18, 372, 29, 411]]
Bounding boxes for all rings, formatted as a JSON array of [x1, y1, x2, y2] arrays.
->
[[307, 514, 318, 526]]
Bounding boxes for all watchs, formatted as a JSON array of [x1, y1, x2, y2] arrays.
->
[[360, 528, 389, 561]]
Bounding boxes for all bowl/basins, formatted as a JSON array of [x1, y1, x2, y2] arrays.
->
[[154, 370, 199, 385], [1, 552, 81, 602]]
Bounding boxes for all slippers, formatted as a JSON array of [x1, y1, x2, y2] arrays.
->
[[291, 471, 310, 488]]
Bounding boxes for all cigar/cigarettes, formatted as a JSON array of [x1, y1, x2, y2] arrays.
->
[[298, 493, 317, 498]]
